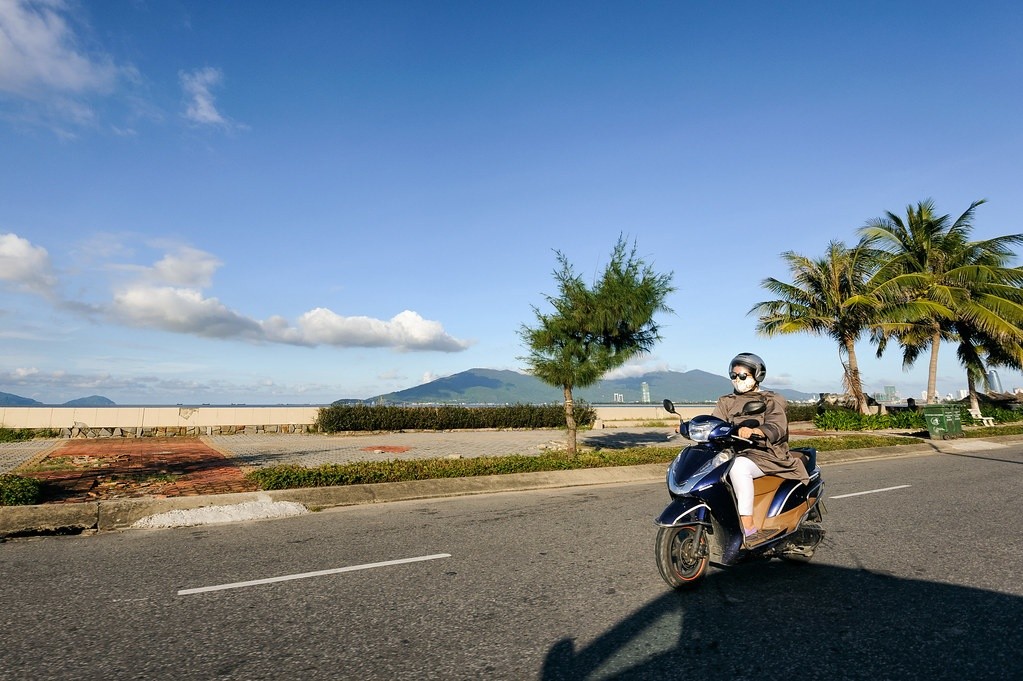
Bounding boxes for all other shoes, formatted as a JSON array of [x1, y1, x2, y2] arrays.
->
[[743, 527, 758, 540]]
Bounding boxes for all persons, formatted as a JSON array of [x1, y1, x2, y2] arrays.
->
[[675, 353, 789, 538]]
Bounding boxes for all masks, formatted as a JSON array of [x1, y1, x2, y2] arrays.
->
[[730, 378, 757, 393]]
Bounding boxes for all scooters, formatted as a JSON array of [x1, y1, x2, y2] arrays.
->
[[653, 398, 829, 592]]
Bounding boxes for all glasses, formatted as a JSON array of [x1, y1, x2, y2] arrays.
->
[[729, 372, 753, 380]]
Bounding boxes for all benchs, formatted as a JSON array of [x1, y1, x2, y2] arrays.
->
[[967, 408, 994, 427]]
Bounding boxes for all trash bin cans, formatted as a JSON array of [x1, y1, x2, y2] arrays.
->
[[924, 403, 965, 439]]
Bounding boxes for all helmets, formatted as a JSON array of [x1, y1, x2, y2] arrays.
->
[[729, 352, 767, 382]]
